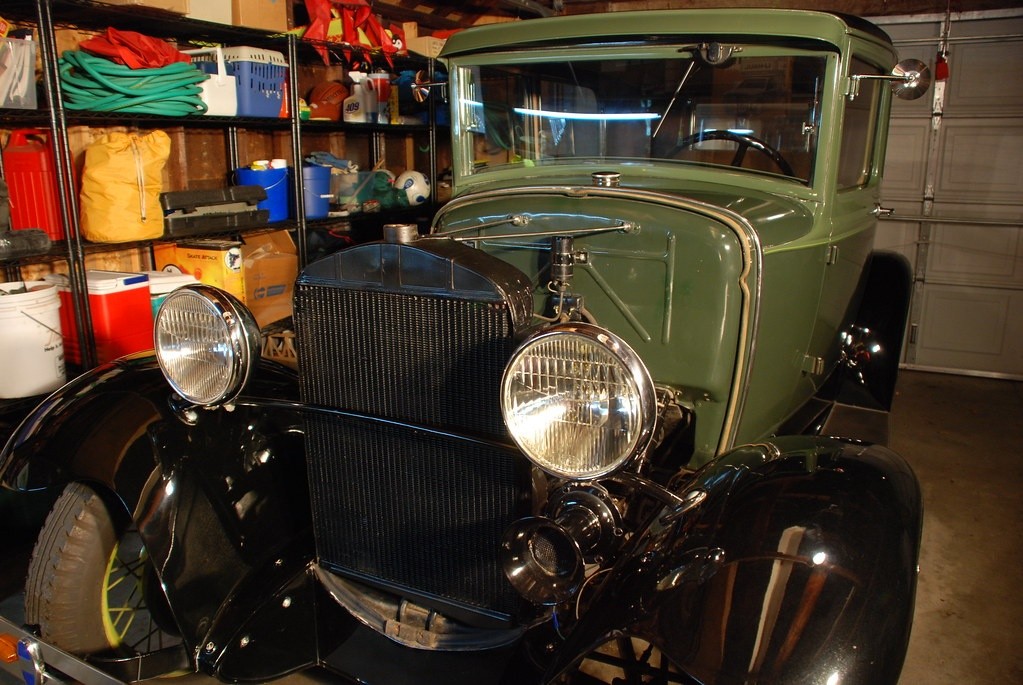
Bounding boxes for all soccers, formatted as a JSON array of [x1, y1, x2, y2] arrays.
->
[[394, 169, 432, 206]]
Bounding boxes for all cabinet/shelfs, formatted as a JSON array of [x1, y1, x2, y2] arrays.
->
[[0, 0, 90, 369], [432, 59, 453, 217], [291, 34, 433, 291], [42, 0, 303, 365]]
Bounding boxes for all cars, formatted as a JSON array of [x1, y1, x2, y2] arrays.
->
[[0, 7, 937, 684]]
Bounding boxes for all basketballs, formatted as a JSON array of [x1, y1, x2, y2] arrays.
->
[[308, 80, 350, 122]]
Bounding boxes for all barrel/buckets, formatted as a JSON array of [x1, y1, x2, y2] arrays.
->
[[0, 281, 66, 398], [288, 165, 334, 219], [231, 159, 289, 223], [2, 128, 77, 240]]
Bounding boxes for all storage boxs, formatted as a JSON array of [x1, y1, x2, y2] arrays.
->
[[39, 268, 158, 369], [240, 229, 297, 329], [184, 46, 287, 116], [140, 269, 202, 314], [0, 37, 36, 109], [408, 33, 448, 58], [178, 240, 246, 312], [158, 184, 268, 234], [327, 170, 375, 209]]
